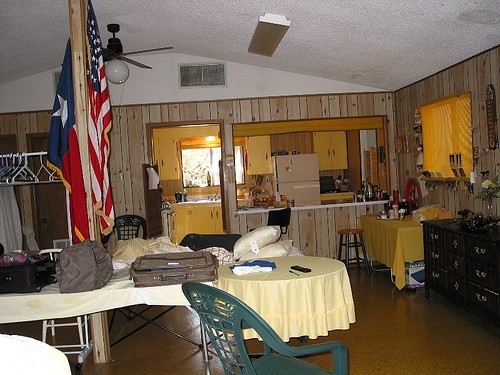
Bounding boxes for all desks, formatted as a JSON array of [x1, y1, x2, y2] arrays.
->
[[219, 256, 356, 358], [0, 246, 304, 364], [360, 214, 424, 290]]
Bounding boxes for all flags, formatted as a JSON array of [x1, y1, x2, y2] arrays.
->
[[46, 0, 115, 245]]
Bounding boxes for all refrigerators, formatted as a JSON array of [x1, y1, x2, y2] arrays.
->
[[271, 154, 321, 207]]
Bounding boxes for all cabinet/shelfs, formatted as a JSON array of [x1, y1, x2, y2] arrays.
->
[[420, 218, 500, 324], [175, 205, 191, 245], [271, 131, 313, 155], [191, 204, 226, 234], [313, 131, 348, 170], [245, 135, 273, 175], [142, 163, 162, 239]]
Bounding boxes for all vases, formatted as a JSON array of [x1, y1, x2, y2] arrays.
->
[[487, 203, 495, 219]]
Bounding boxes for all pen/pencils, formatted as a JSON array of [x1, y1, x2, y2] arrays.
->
[[289, 270, 300, 277]]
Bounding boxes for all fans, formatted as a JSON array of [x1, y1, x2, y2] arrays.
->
[[101, 24, 173, 69]]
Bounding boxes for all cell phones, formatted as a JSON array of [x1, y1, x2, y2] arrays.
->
[[291, 266, 311, 272]]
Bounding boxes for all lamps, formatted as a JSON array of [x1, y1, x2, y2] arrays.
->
[[105, 57, 129, 84], [247, 14, 292, 58]]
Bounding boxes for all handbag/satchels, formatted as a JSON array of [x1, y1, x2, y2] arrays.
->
[[129, 251, 215, 287]]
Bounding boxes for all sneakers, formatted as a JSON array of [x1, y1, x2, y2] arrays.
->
[[55, 239, 113, 293]]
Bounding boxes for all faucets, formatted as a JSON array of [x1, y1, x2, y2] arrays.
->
[[209, 191, 220, 201]]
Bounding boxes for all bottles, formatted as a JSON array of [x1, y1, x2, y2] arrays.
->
[[207, 171, 211, 187], [357, 180, 388, 202], [271, 149, 301, 156], [379, 185, 419, 219]]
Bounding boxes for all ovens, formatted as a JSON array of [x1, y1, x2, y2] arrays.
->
[[321, 193, 354, 204]]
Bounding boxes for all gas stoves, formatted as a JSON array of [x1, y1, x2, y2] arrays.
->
[[320, 176, 350, 194]]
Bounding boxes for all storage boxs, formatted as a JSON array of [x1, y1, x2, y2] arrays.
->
[[405, 267, 425, 288]]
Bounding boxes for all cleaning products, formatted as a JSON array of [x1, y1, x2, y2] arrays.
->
[[207, 171, 211, 186]]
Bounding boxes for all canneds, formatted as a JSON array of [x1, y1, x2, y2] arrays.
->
[[280, 194, 285, 202], [287, 198, 294, 207]]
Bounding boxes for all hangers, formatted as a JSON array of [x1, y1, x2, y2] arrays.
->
[[0, 151, 62, 186]]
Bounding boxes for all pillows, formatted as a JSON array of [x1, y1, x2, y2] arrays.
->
[[234, 225, 293, 260]]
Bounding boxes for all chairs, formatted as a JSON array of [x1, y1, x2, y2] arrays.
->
[[182, 282, 349, 375], [40, 249, 88, 355], [249, 207, 291, 234], [115, 215, 148, 239]]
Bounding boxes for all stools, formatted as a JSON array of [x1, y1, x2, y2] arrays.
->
[[337, 229, 370, 274]]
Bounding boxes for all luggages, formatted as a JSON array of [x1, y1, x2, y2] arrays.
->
[[0, 243, 57, 293]]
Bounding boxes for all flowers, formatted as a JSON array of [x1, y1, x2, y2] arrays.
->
[[474, 176, 500, 207]]
